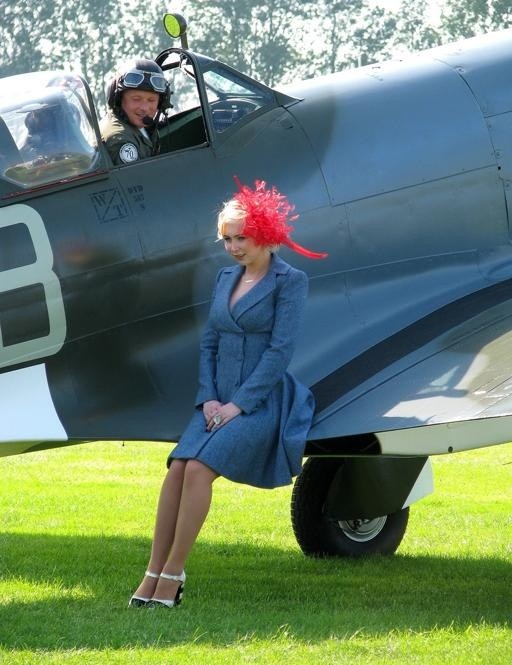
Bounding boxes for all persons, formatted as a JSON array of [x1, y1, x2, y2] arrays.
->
[[88, 57, 172, 171], [126, 197, 316, 611], [16, 86, 93, 161]]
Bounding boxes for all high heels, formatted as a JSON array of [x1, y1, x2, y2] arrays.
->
[[127, 567, 187, 610]]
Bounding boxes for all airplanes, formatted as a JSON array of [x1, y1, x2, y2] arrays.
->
[[0, 28, 512, 559]]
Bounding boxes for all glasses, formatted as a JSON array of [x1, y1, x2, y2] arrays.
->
[[115, 70, 167, 93]]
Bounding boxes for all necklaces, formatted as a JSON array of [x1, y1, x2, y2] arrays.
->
[[239, 270, 268, 283]]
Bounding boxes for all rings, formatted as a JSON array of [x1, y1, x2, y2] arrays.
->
[[213, 415, 221, 424]]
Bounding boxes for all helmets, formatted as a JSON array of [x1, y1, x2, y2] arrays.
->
[[107, 58, 165, 106]]
